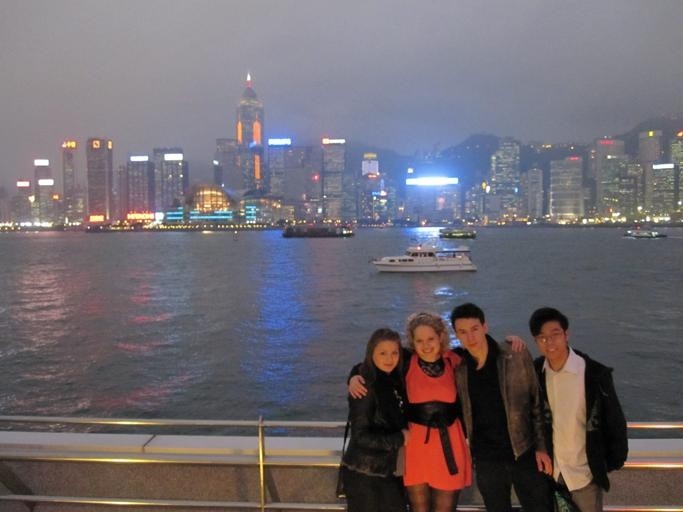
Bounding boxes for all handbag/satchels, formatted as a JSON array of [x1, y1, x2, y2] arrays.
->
[[336, 464, 346, 498]]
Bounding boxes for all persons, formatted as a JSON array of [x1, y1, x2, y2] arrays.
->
[[450, 305, 554, 512], [348, 310, 527, 512], [529, 307, 629, 512], [341, 329, 412, 512]]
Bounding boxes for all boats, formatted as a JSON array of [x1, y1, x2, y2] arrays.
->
[[439, 228, 478, 238], [372, 238, 478, 272], [282, 225, 355, 238], [623, 230, 667, 238]]
